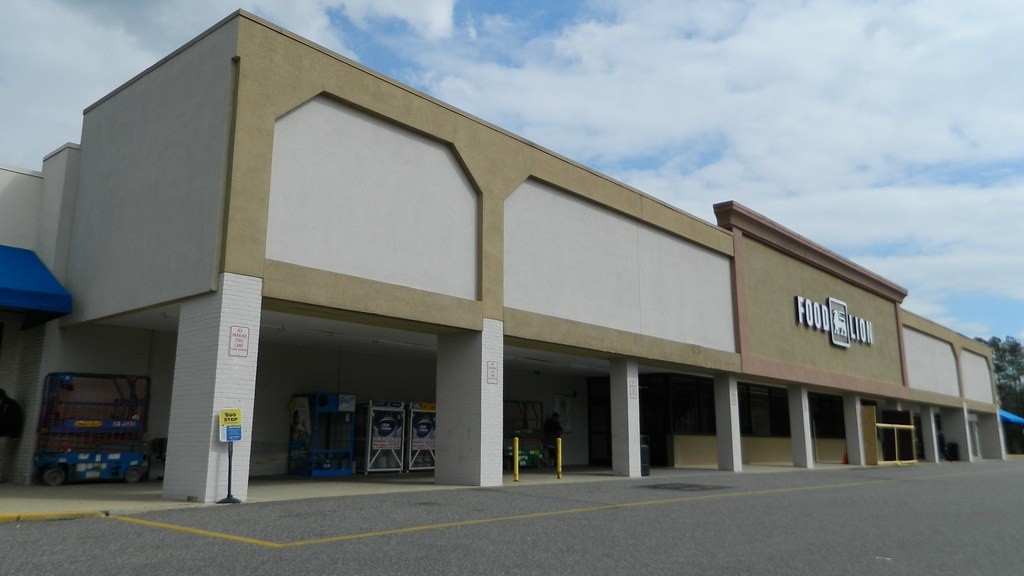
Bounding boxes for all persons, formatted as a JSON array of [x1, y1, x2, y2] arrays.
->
[[0, 387, 22, 451], [542, 412, 563, 469], [290, 410, 308, 440], [938, 431, 952, 460]]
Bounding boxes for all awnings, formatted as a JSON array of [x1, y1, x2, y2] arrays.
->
[[999, 409, 1024, 424]]
